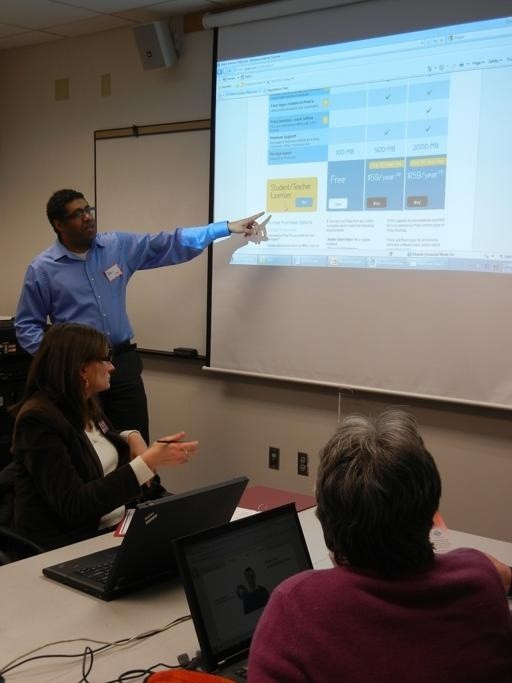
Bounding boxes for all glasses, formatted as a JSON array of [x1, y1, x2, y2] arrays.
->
[[68, 205, 91, 218], [96, 346, 112, 361]]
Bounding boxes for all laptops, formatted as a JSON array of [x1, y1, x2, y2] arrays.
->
[[174, 502, 313, 683], [42, 477, 249, 602]]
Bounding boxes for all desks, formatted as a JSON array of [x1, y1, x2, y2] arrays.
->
[[0, 485, 511, 682]]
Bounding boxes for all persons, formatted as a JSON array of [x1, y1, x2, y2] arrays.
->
[[247, 406, 512, 682], [238, 569, 271, 613], [12, 322, 201, 553], [16, 189, 266, 438]]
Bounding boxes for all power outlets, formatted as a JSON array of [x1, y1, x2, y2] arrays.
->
[[298, 452, 307, 476], [269, 447, 279, 469]]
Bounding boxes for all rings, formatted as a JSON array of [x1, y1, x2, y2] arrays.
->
[[183, 449, 188, 455]]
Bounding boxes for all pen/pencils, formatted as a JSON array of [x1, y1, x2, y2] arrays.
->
[[157, 440, 185, 444]]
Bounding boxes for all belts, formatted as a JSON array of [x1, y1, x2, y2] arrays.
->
[[112, 339, 137, 353]]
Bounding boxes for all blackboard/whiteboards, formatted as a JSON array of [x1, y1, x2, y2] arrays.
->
[[89, 117, 211, 362]]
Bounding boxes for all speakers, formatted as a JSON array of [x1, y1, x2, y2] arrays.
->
[[132, 20, 178, 74]]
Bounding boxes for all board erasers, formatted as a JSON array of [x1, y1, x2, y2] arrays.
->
[[172, 349, 199, 360]]
